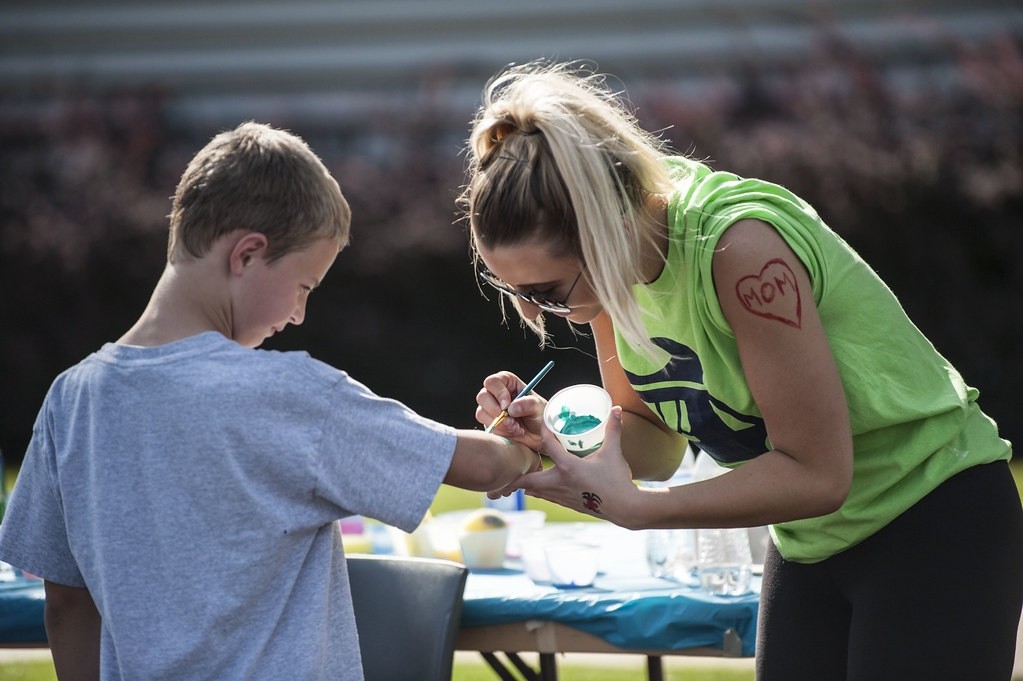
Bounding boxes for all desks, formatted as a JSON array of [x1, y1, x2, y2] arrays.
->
[[0, 520, 769, 681]]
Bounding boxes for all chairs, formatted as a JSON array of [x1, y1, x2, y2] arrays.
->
[[345, 553, 468, 681]]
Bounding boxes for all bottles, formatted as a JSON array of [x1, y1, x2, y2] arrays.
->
[[641, 439, 770, 597]]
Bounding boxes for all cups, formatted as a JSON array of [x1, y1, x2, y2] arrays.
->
[[458, 511, 640, 592], [543, 384, 613, 458]]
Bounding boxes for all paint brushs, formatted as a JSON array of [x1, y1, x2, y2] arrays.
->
[[481, 359, 557, 434]]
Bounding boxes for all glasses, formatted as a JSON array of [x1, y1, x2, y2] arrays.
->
[[480, 262, 587, 312]]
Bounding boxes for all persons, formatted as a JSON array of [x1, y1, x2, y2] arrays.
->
[[0, 120, 542, 680], [470, 61, 1023, 680]]
